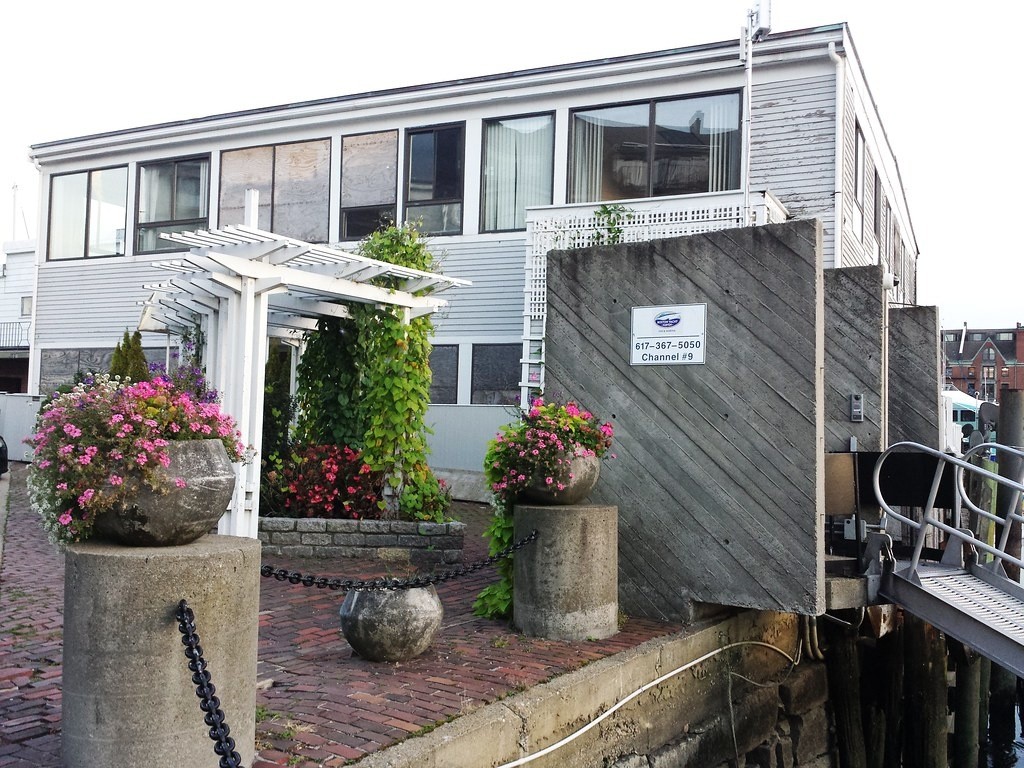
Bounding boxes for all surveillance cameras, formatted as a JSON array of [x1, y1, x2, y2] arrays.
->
[[883, 273, 899, 290]]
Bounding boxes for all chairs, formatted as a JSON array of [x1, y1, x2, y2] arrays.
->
[[824, 451, 863, 573]]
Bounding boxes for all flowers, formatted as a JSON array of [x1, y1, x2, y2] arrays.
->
[[22, 339, 260, 554], [470, 362, 618, 621]]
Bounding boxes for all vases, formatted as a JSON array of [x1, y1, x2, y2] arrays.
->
[[523, 443, 600, 505], [93, 436, 237, 544]]
